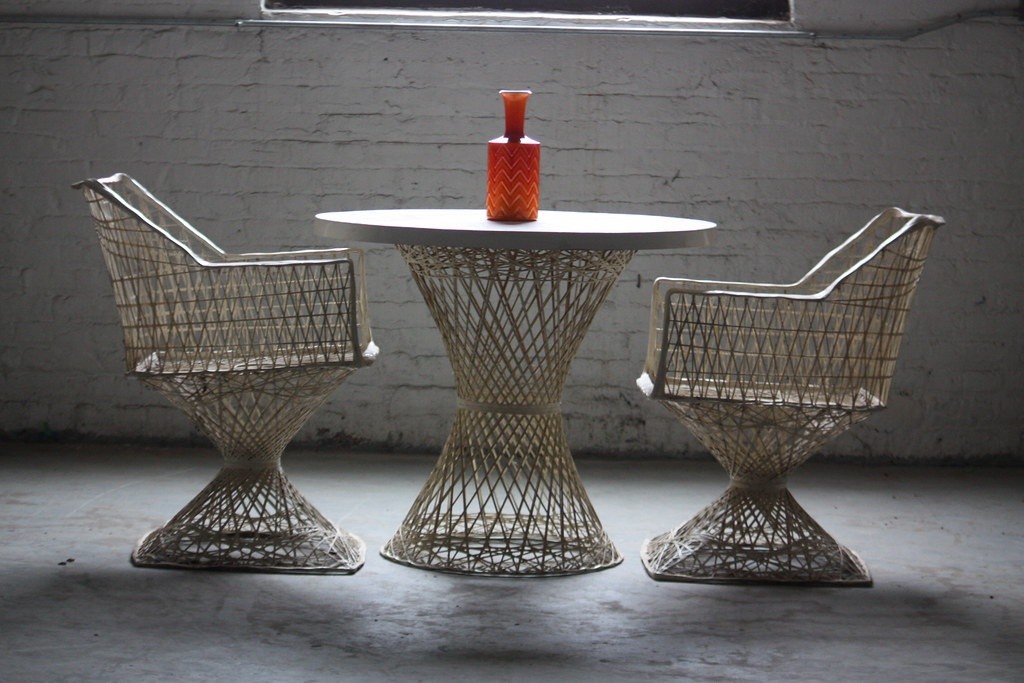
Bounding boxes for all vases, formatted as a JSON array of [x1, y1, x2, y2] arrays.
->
[[487, 89, 540, 220]]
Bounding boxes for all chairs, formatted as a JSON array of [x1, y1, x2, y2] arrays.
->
[[635, 205, 946, 585], [69, 170, 379, 576]]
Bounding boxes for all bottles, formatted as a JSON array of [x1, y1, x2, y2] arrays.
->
[[487, 90, 541, 221]]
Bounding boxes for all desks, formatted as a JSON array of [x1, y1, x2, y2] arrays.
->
[[316, 209, 717, 576]]
